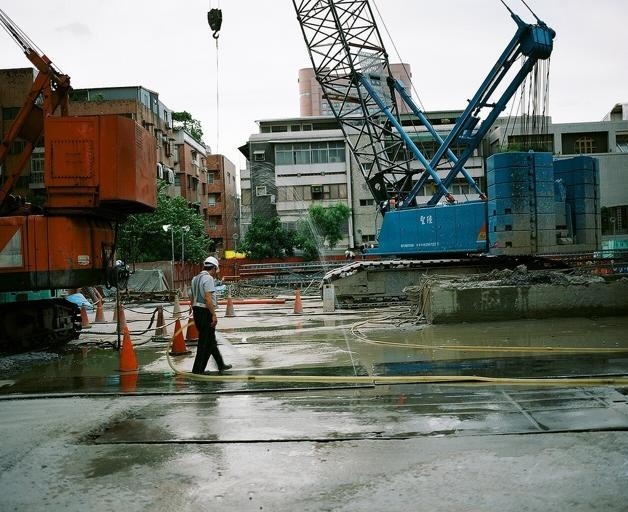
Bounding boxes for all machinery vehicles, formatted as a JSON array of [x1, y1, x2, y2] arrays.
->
[[0, 8, 158, 349], [292, 0, 603, 309]]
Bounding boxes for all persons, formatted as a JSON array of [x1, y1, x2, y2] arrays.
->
[[189, 255, 233, 375]]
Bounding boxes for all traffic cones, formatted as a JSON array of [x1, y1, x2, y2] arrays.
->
[[80, 300, 137, 372], [289, 292, 307, 317], [151, 294, 236, 356]]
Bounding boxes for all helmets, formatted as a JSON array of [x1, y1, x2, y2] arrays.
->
[[203, 256, 220, 274]]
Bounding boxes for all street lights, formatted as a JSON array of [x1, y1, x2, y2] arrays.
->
[[162, 224, 190, 266]]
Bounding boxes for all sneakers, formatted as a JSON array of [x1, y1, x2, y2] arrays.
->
[[219, 364, 232, 372]]
